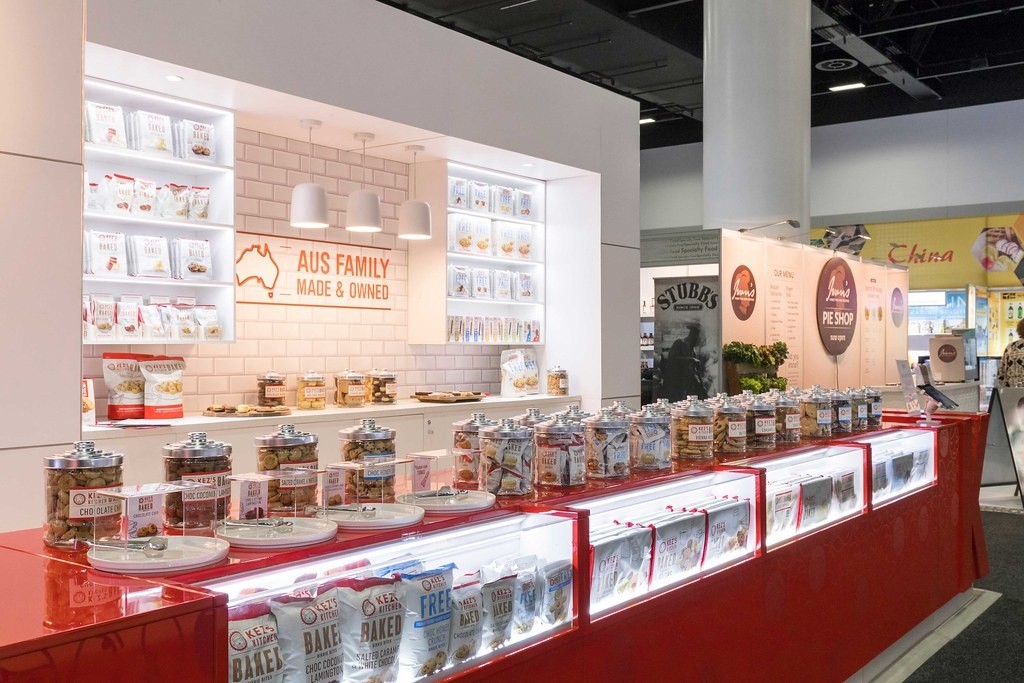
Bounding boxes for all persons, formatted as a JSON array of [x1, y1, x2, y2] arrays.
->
[[996, 318, 1024, 388]]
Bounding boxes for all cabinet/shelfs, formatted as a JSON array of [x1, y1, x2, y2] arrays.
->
[[407, 159, 547, 347], [0, 408, 962, 683], [641, 313, 655, 351], [725, 360, 779, 397], [81, 393, 590, 519], [82, 74, 237, 345]]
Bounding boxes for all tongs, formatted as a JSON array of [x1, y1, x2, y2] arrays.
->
[[304, 505, 377, 517], [85, 537, 169, 557], [409, 485, 469, 502], [222, 516, 292, 528]]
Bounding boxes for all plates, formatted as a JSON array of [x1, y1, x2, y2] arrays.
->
[[395, 489, 497, 514], [214, 517, 339, 549], [315, 503, 426, 530], [87, 536, 230, 574]]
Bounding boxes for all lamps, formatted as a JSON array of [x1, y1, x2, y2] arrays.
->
[[739, 220, 801, 232], [289, 120, 330, 228], [817, 233, 871, 248], [776, 227, 839, 240], [396, 145, 430, 239], [346, 133, 383, 233]]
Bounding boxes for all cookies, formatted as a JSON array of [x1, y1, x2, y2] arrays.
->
[[206, 376, 482, 412], [42, 440, 396, 544], [673, 396, 883, 459]]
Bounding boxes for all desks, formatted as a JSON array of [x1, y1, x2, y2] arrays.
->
[[865, 380, 983, 414]]
